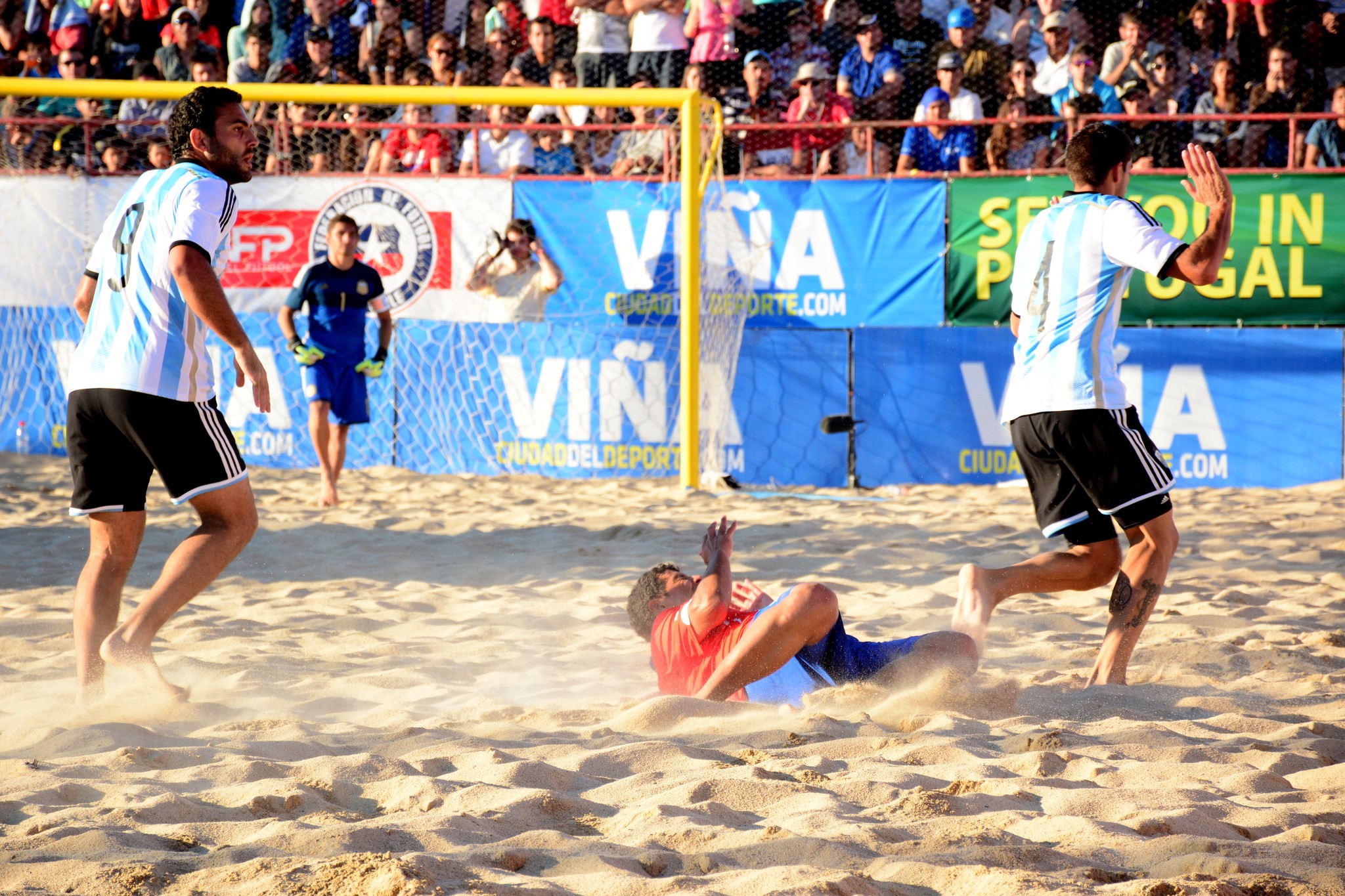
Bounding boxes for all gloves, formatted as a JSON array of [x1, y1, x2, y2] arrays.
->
[[288, 335, 325, 366], [354, 346, 388, 378]]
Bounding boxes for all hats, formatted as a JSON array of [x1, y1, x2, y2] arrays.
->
[[937, 51, 963, 70], [172, 6, 201, 26], [743, 49, 776, 68], [787, 6, 813, 22], [852, 13, 882, 34], [922, 85, 950, 114], [789, 62, 836, 90], [1040, 10, 1071, 32], [946, 6, 975, 28], [1117, 78, 1149, 101], [303, 25, 330, 43]]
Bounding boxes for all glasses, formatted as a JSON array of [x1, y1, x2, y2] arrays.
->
[[59, 12, 1181, 123]]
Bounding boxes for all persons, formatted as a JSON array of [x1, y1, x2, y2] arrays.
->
[[3, 0, 1345, 178], [276, 214, 393, 505], [467, 217, 566, 324], [952, 124, 1237, 688], [68, 88, 271, 707], [625, 518, 976, 703]]
